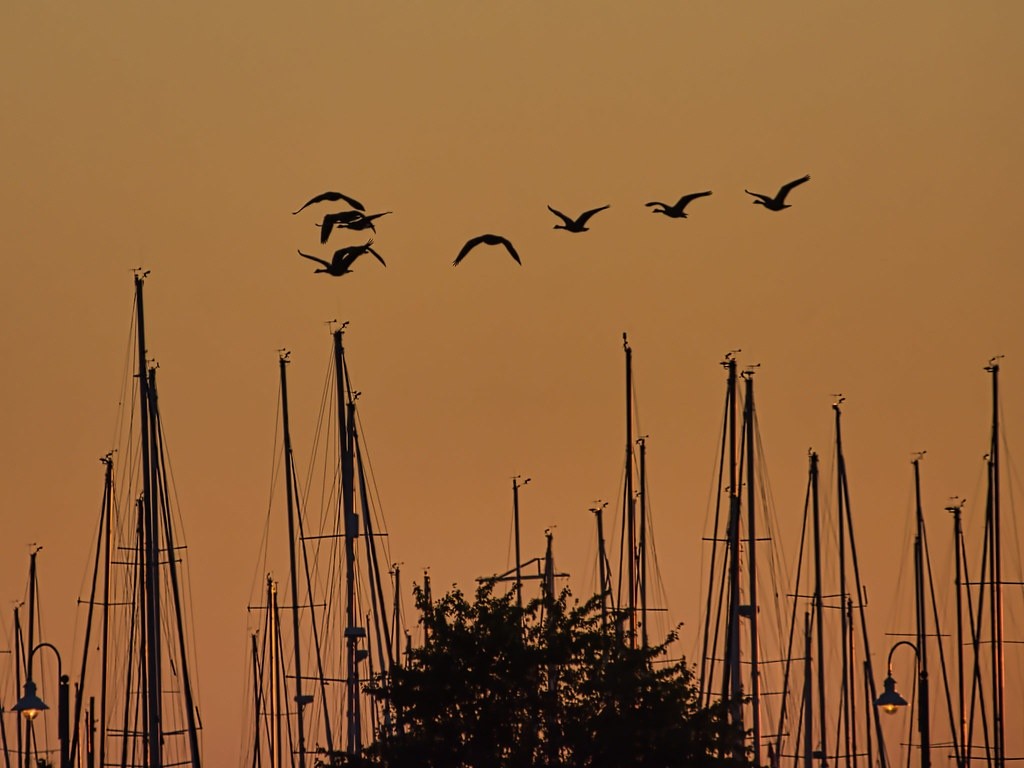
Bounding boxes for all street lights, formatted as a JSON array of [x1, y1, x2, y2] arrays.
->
[[9, 642, 70, 768], [873, 640, 932, 768]]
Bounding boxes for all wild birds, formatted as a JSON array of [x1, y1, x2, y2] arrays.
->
[[292, 192, 366, 215], [452, 234, 523, 267], [744, 173, 810, 211], [644, 190, 713, 219], [547, 204, 611, 233], [297, 238, 387, 277], [337, 211, 394, 230], [315, 210, 377, 244]]
